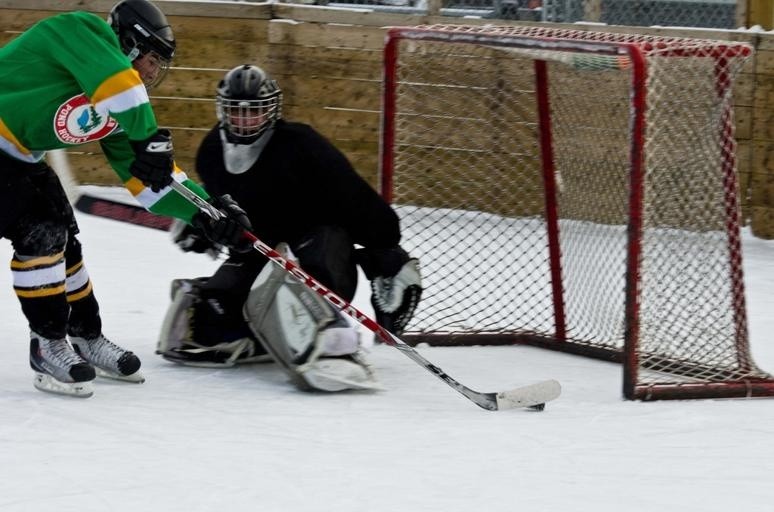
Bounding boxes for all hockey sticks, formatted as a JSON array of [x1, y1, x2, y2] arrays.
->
[[168, 179, 561, 411]]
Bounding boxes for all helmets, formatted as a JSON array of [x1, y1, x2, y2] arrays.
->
[[107, 0, 176, 95], [215, 64, 284, 175]]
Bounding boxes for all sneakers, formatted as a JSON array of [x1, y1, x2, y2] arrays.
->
[[69, 331, 141, 378], [29, 327, 97, 383]]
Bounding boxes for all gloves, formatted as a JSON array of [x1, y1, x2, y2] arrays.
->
[[128, 127, 175, 192], [192, 193, 254, 251]]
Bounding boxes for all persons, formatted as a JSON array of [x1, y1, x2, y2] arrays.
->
[[152, 65, 424, 393], [0, 0, 254, 385]]
